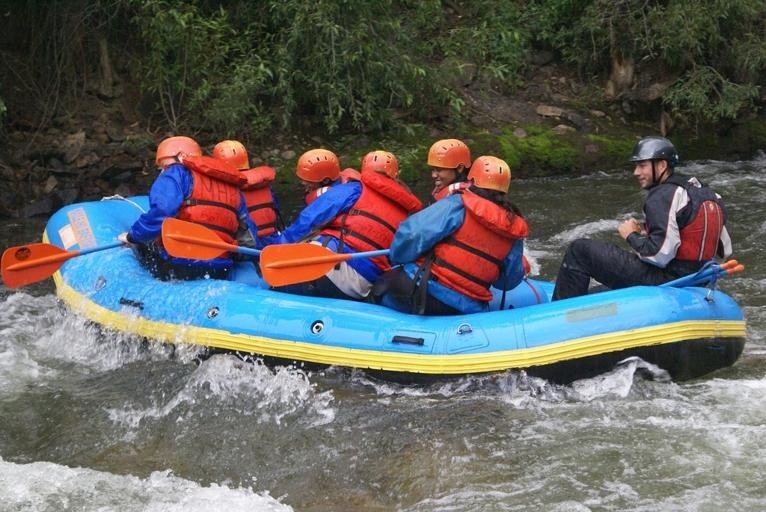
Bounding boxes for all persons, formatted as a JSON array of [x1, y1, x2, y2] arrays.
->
[[115, 136, 254, 282], [214, 141, 279, 256], [425, 139, 473, 206], [261, 149, 421, 302], [387, 158, 533, 315], [548, 133, 734, 305], [293, 148, 359, 205]]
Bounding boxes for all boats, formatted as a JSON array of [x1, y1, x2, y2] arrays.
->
[[40, 193, 745, 384]]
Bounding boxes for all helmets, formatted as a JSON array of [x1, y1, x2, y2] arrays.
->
[[211, 139, 252, 172], [296, 149, 342, 184], [427, 138, 473, 171], [155, 135, 204, 167], [628, 134, 679, 167], [361, 149, 400, 182], [468, 154, 512, 194]]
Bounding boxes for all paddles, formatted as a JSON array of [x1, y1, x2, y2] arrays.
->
[[162, 216, 264, 261], [2, 238, 126, 289], [260, 247, 391, 286]]
